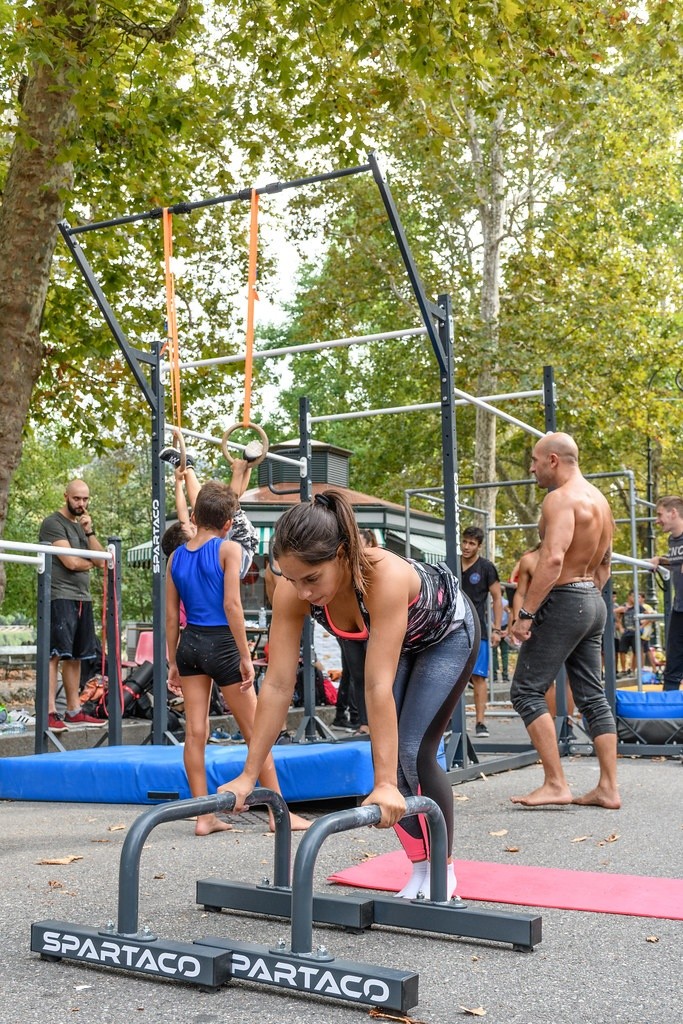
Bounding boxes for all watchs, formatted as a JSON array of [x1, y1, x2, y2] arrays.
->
[[518, 608, 536, 620]]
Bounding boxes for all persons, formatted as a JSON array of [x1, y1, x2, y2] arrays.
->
[[264, 559, 330, 706], [165, 484, 313, 836], [491, 586, 511, 682], [39, 478, 107, 732], [461, 526, 503, 737], [601, 590, 659, 681], [161, 458, 260, 580], [505, 550, 577, 741], [509, 432, 621, 809], [644, 496, 683, 692], [218, 489, 480, 901]]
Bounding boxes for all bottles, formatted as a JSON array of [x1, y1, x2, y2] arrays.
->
[[0, 721, 26, 736], [258, 607, 267, 628], [258, 673, 264, 689]]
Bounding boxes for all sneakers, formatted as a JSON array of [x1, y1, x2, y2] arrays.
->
[[243, 439, 263, 462], [475, 723, 489, 737], [46, 711, 71, 732], [209, 726, 231, 743], [443, 719, 453, 734], [159, 446, 193, 468], [63, 708, 108, 727], [230, 730, 246, 743], [8, 710, 37, 727]]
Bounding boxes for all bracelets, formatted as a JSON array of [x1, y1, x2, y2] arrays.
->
[[85, 531, 95, 537], [493, 628, 500, 632]]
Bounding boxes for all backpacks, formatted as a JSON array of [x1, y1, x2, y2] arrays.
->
[[80, 675, 110, 715], [295, 666, 326, 707], [323, 678, 338, 706]]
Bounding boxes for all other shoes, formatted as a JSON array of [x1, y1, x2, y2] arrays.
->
[[332, 719, 348, 730], [617, 671, 627, 678], [494, 676, 497, 681], [346, 722, 361, 732], [502, 677, 508, 683]]
